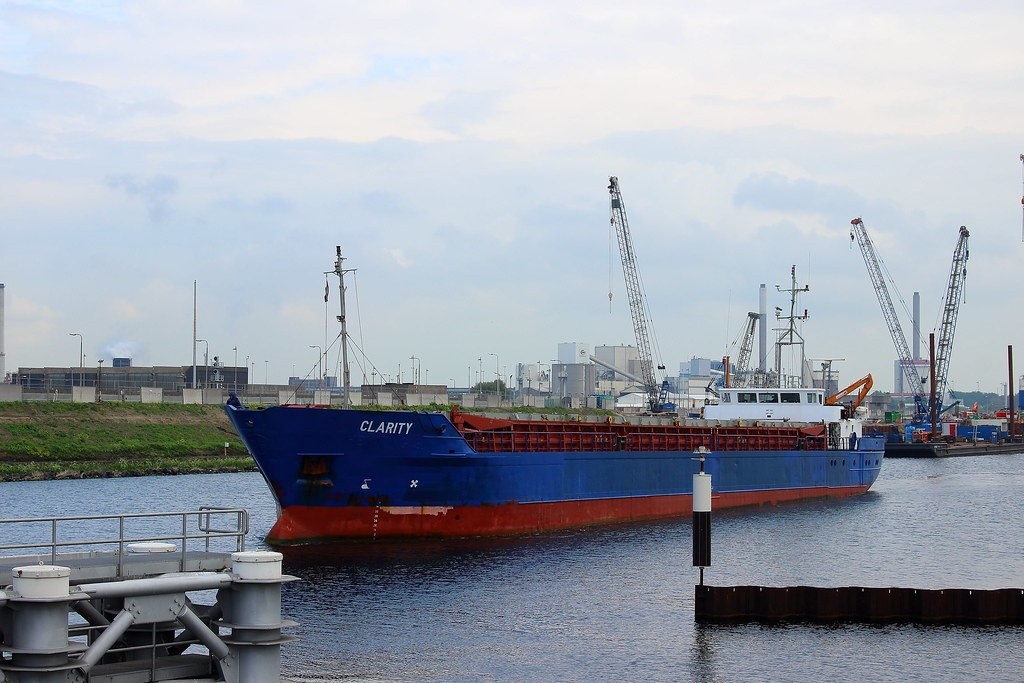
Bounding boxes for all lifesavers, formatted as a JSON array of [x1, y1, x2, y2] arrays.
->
[[578, 415, 582, 422], [738, 421, 743, 427], [609, 416, 613, 423]]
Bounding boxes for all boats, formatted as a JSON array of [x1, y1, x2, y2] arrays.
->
[[219, 245, 887, 551]]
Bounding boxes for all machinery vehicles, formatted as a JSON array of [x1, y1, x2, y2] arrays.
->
[[849, 216, 971, 445], [606, 175, 701, 419]]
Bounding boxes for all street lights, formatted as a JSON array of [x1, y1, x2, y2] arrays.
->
[[499, 374, 507, 400], [232, 346, 238, 394], [538, 383, 543, 396], [491, 353, 498, 395], [83, 354, 86, 386], [550, 359, 562, 400], [97, 359, 104, 402], [251, 362, 255, 395], [539, 363, 551, 397], [69, 334, 82, 387], [195, 339, 209, 389], [265, 360, 269, 384], [308, 345, 485, 394], [293, 365, 295, 377], [246, 356, 250, 368], [509, 374, 512, 400]]
